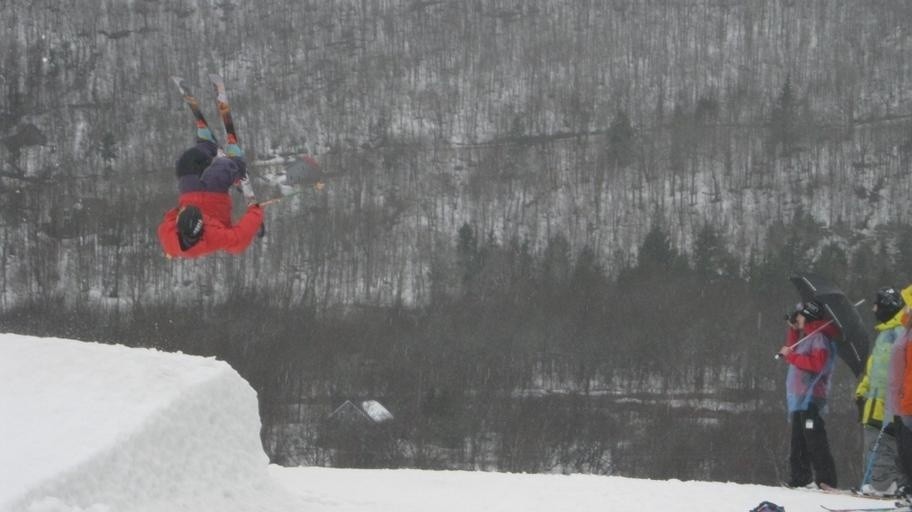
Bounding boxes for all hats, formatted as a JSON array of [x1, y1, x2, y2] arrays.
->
[[176, 206, 205, 251], [795, 300, 822, 322], [874, 286, 903, 314]]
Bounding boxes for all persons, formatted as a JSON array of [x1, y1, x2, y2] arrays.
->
[[156, 120, 263, 258], [850, 285, 912, 508], [778, 300, 841, 489]]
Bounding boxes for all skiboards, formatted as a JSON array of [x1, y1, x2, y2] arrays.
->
[[169, 72, 266, 240], [818, 502, 911, 512]]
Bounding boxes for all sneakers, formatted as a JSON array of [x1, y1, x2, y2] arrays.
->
[[861, 481, 898, 496]]
